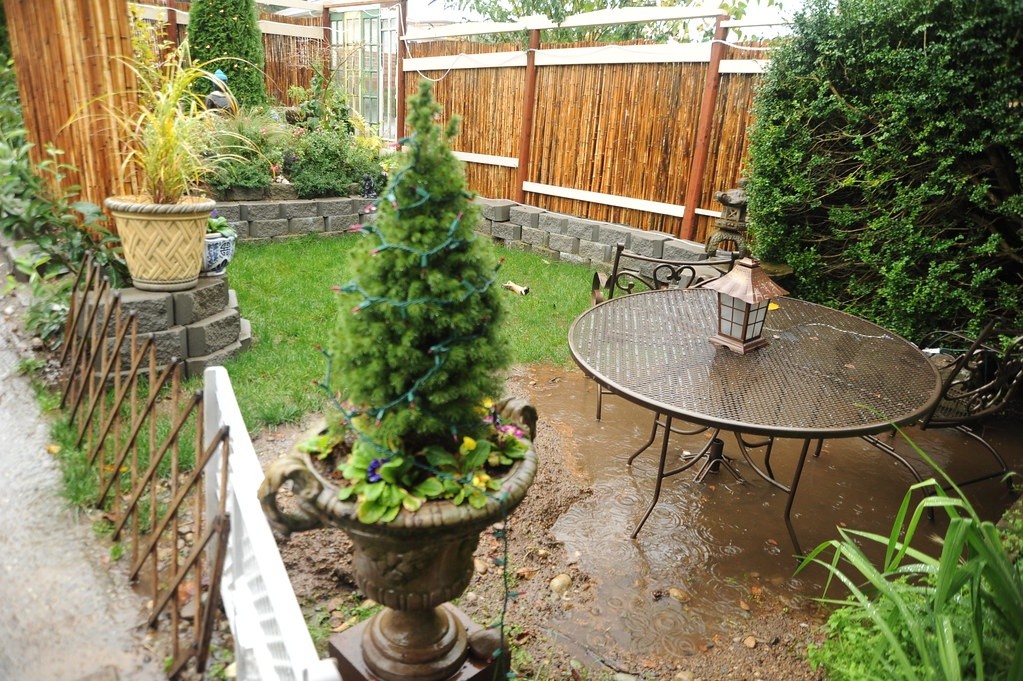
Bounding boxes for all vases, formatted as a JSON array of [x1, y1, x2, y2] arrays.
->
[[258, 397, 538, 681]]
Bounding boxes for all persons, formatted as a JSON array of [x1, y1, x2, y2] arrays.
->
[[202, 69, 239, 115]]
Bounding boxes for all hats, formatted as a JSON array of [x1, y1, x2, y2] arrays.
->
[[214, 69, 227, 81]]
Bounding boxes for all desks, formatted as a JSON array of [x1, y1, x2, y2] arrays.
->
[[567, 288, 942, 539]]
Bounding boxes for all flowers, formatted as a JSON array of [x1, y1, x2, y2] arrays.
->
[[299, 77, 529, 525]]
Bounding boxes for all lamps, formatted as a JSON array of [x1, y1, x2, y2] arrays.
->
[[703, 256, 790, 354]]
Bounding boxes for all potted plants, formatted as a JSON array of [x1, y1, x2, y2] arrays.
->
[[51, 0, 282, 293]]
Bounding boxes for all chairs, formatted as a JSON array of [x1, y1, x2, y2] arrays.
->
[[591, 242, 739, 420], [812, 317, 1023, 522]]
